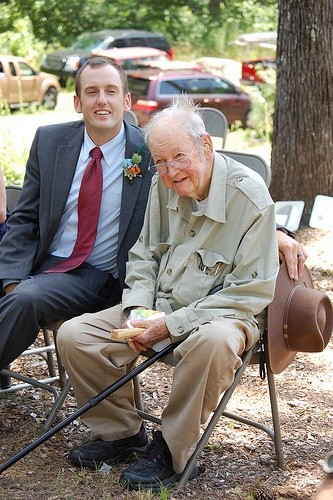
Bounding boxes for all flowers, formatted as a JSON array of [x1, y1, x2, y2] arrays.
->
[[121, 153, 143, 184]]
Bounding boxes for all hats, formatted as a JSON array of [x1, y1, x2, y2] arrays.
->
[[266, 260, 333, 373]]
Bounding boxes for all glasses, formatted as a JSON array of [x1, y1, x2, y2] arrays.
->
[[147, 139, 201, 177]]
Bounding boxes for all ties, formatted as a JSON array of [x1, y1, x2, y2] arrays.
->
[[41, 147, 103, 273]]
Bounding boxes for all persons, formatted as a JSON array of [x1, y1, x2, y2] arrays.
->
[[0, 56, 308, 372], [55, 104, 279, 492]]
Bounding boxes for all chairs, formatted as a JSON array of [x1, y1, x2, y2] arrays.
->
[[0, 107, 283, 500]]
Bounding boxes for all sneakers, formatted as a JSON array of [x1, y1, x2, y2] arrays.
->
[[68, 420, 150, 470], [119, 429, 199, 492]]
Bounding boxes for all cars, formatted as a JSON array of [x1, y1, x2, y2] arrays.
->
[[41, 29, 252, 127], [0, 55, 61, 109]]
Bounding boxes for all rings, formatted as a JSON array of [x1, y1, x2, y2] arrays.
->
[[128, 338, 134, 342], [297, 253, 303, 257]]
